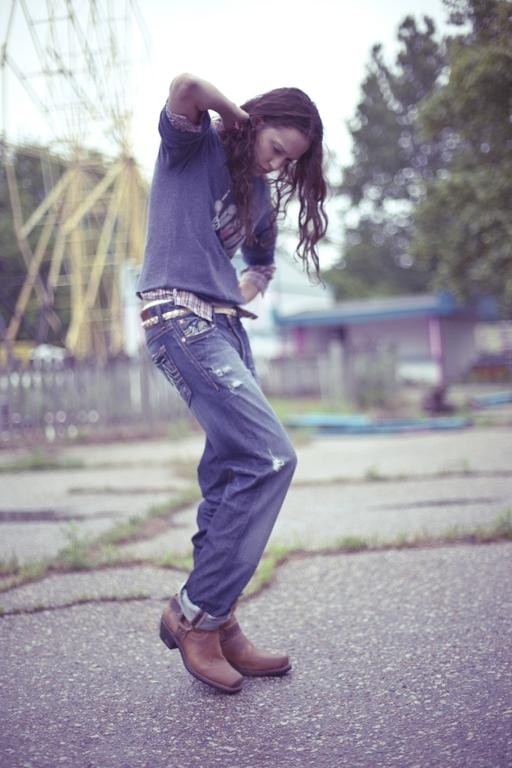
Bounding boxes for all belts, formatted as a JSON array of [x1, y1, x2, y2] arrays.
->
[[141, 299, 258, 323]]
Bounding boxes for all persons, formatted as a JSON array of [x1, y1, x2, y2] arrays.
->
[[137, 73, 328, 693]]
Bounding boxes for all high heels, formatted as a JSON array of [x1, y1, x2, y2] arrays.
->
[[219, 611, 292, 677], [159, 592, 242, 693]]
